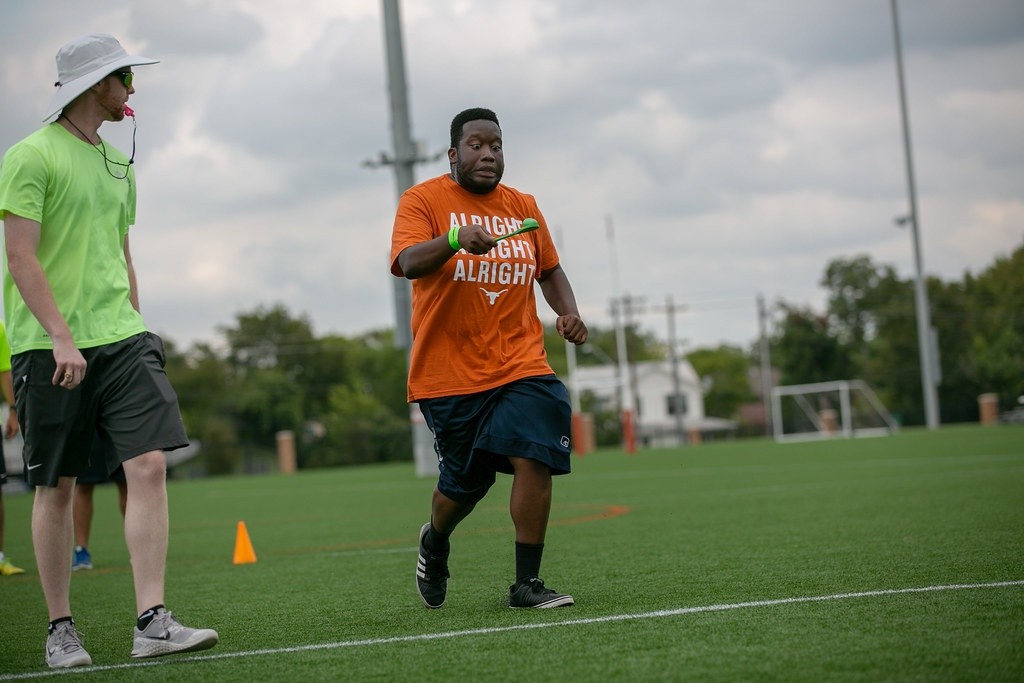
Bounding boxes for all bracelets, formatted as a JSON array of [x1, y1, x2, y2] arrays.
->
[[447, 227, 464, 252], [10, 405, 17, 412]]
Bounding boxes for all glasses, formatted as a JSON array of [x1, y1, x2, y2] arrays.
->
[[114, 71, 132, 86]]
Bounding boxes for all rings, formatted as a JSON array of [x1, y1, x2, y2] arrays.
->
[[64, 372, 74, 380]]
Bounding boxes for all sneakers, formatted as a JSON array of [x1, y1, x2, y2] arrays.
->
[[46, 625, 91, 669], [72, 546, 92, 569], [416, 522, 451, 608], [509, 584, 575, 610], [0, 561, 24, 576], [131, 613, 217, 657]]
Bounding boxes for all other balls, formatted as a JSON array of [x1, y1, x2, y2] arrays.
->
[[523, 218, 538, 227]]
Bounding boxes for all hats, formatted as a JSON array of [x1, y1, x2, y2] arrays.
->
[[42, 34, 160, 122]]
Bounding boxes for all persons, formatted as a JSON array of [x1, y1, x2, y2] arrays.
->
[[389, 107, 586, 611], [0, 35, 220, 671], [0, 320, 25, 576], [70, 462, 127, 574]]
